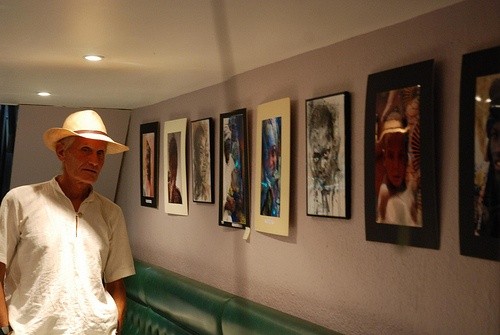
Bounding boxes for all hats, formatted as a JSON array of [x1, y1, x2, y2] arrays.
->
[[43, 110, 130, 155]]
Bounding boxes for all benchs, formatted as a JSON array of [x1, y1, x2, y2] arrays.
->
[[121, 260, 347, 335]]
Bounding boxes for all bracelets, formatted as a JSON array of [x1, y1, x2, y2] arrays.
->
[[0, 326, 12, 335]]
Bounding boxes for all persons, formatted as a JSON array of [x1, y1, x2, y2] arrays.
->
[[0, 110, 136, 335], [378, 112, 410, 219]]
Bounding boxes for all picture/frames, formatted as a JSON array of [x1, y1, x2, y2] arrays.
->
[[218, 108, 250, 231], [253, 97, 290, 237], [305, 90, 352, 219], [163, 117, 189, 216], [139, 121, 160, 208], [188, 117, 214, 204], [365, 57, 440, 251], [458, 44, 500, 263]]
